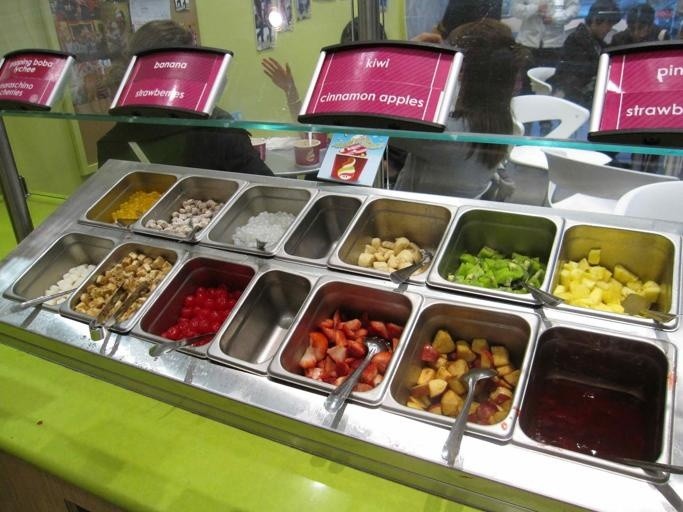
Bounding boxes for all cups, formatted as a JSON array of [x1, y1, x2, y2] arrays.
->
[[304, 131, 327, 148], [254, 143, 266, 160], [293, 139, 321, 166], [330, 153, 367, 183]]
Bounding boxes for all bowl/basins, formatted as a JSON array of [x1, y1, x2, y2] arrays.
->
[[425, 204, 566, 306], [61, 238, 187, 334], [207, 261, 323, 375], [268, 276, 424, 407], [539, 220, 682, 332], [380, 297, 541, 444], [78, 169, 182, 228], [132, 252, 259, 359], [327, 192, 459, 284], [2, 228, 120, 313], [512, 318, 666, 483], [199, 184, 320, 255], [130, 174, 248, 243], [274, 190, 368, 267]]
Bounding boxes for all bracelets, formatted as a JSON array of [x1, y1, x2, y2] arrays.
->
[[286, 97, 301, 106]]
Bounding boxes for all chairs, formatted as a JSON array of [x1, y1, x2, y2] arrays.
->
[[505, 95, 613, 206], [526, 65, 571, 97], [534, 149, 682, 222], [616, 190, 683, 230]]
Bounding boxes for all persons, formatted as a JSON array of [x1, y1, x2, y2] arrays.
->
[[429, 1, 502, 40], [508, 0, 581, 68], [388, 20, 522, 201], [555, 1, 622, 91], [611, 3, 669, 48], [96, 20, 276, 180], [262, 15, 388, 126]]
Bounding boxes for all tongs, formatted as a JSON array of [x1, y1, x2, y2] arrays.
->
[[88, 282, 150, 341]]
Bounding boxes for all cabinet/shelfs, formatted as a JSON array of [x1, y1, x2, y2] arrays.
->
[[0, 107, 683, 511]]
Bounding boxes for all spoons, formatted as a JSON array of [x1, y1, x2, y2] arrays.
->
[[501, 278, 566, 306], [149, 332, 217, 357], [622, 293, 676, 325], [442, 368, 500, 462], [391, 249, 435, 284], [11, 289, 78, 312], [323, 338, 387, 411]]
